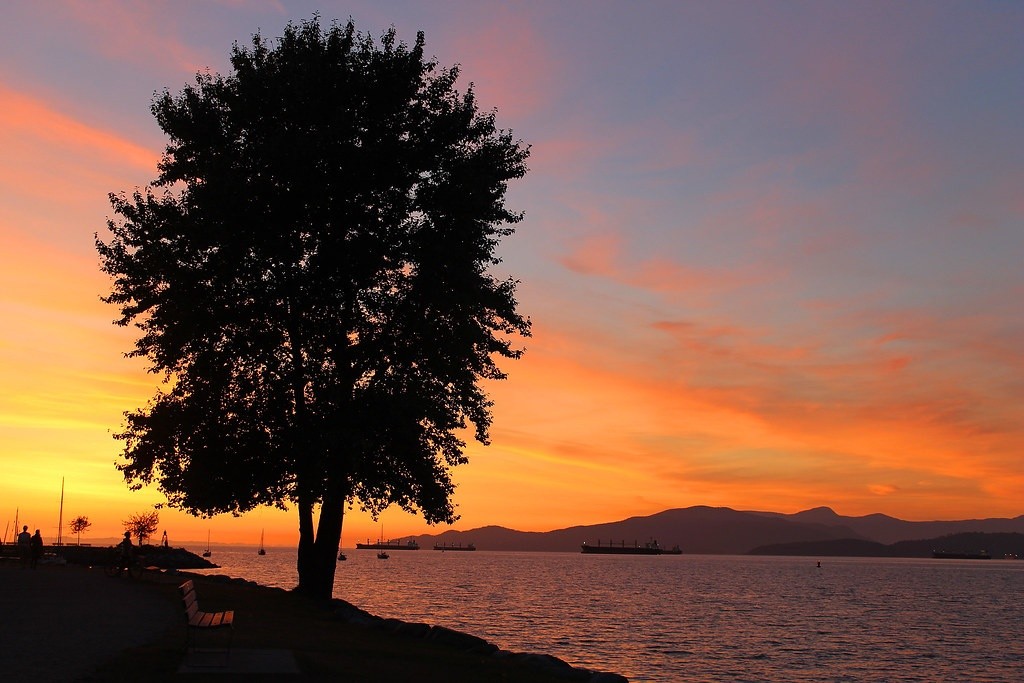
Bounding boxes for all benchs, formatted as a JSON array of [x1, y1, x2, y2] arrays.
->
[[0, 556, 169, 584], [177, 579, 235, 669]]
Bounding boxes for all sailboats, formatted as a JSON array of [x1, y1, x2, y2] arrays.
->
[[203, 529, 212, 556], [258, 528, 265, 554], [338, 538, 347, 560], [377, 523, 390, 559]]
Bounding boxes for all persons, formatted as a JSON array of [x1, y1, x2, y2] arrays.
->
[[116, 532, 133, 578], [18, 525, 30, 569], [30, 529, 43, 569]]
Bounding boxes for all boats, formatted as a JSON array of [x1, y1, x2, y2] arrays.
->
[[581, 536, 682, 554], [356, 538, 420, 550], [933, 544, 991, 559], [433, 541, 477, 550]]
